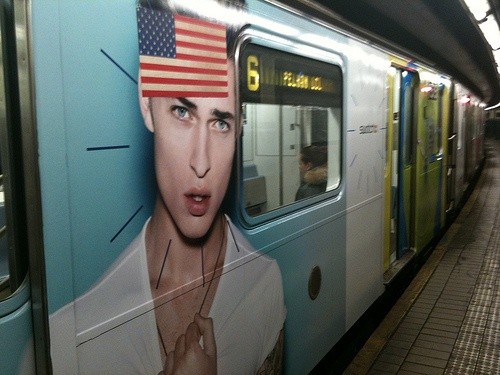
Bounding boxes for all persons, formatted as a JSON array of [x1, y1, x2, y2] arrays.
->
[[295, 145, 329, 201], [50, 0, 291, 375]]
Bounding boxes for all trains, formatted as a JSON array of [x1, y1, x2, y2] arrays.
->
[[1, 0, 486, 375]]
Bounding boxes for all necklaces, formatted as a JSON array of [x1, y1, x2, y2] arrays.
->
[[155, 222, 228, 360]]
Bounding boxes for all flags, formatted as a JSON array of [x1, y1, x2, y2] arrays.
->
[[136, 7, 230, 97]]
